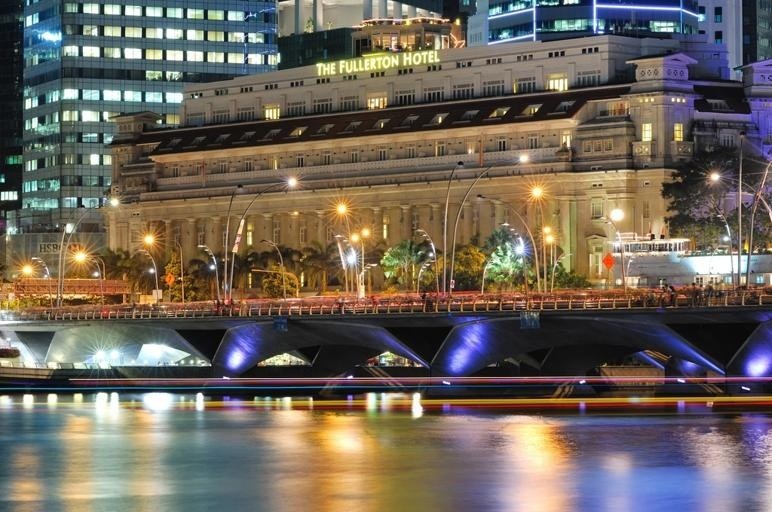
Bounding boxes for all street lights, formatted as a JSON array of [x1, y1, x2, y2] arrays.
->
[[692, 169, 772, 296], [16, 193, 123, 308], [137, 231, 221, 308], [285, 199, 373, 294], [223, 175, 301, 304], [476, 177, 559, 293], [438, 152, 535, 297], [598, 207, 627, 301]]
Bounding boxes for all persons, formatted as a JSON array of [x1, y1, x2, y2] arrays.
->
[[212, 298, 249, 317], [371, 295, 378, 313], [423, 291, 452, 304], [646, 277, 725, 306]]
